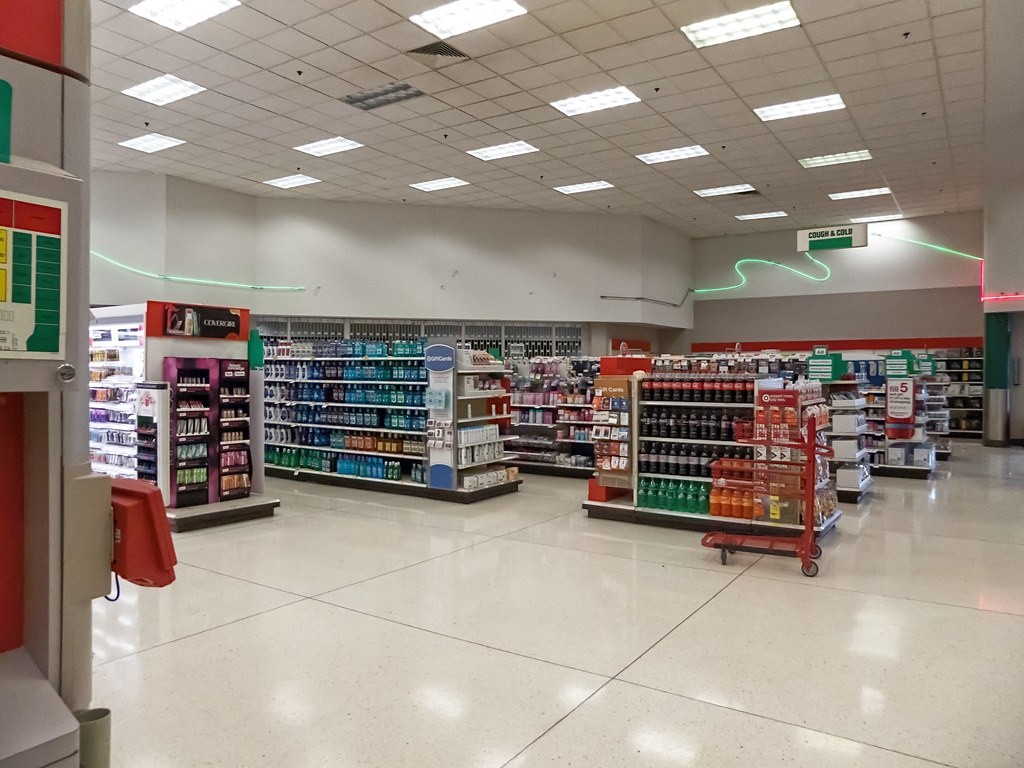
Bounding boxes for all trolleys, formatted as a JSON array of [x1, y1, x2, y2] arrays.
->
[[701, 411, 835, 578]]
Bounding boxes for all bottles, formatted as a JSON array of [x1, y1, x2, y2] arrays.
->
[[185, 309, 194, 336], [637, 477, 753, 519], [637, 442, 754, 480], [802, 404, 829, 426], [783, 378, 822, 401], [639, 407, 754, 441], [262, 360, 429, 385], [642, 370, 755, 403], [261, 336, 427, 359], [263, 381, 427, 486]]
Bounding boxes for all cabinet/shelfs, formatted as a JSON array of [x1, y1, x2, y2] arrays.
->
[[88, 301, 983, 540]]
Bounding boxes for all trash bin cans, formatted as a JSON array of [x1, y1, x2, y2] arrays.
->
[[983, 388, 1010, 448]]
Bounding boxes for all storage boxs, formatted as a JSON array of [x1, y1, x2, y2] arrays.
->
[[832, 397, 867, 407], [888, 445, 907, 466], [896, 426, 924, 441], [836, 464, 871, 488], [832, 436, 865, 459], [970, 385, 983, 395], [914, 444, 936, 467], [832, 410, 866, 433]]
